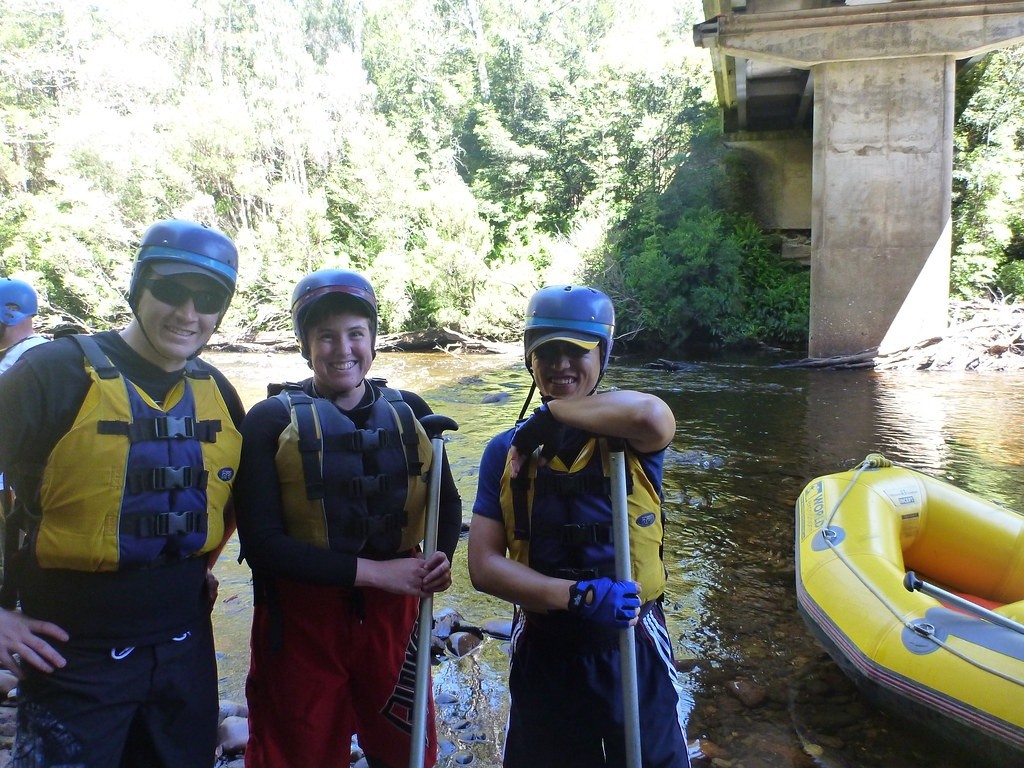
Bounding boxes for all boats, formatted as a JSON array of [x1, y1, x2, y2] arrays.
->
[[795, 453, 1024, 767]]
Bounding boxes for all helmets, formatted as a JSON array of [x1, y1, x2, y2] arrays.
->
[[0, 278, 38, 324], [292, 268, 377, 340], [129, 218, 238, 333], [524, 285, 616, 377]]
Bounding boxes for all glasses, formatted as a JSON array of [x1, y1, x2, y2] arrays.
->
[[141, 275, 228, 315]]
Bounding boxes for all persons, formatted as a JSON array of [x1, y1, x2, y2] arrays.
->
[[467, 287, 692, 767], [1, 219, 247, 768], [1, 272, 50, 708], [233, 268, 464, 768]]
